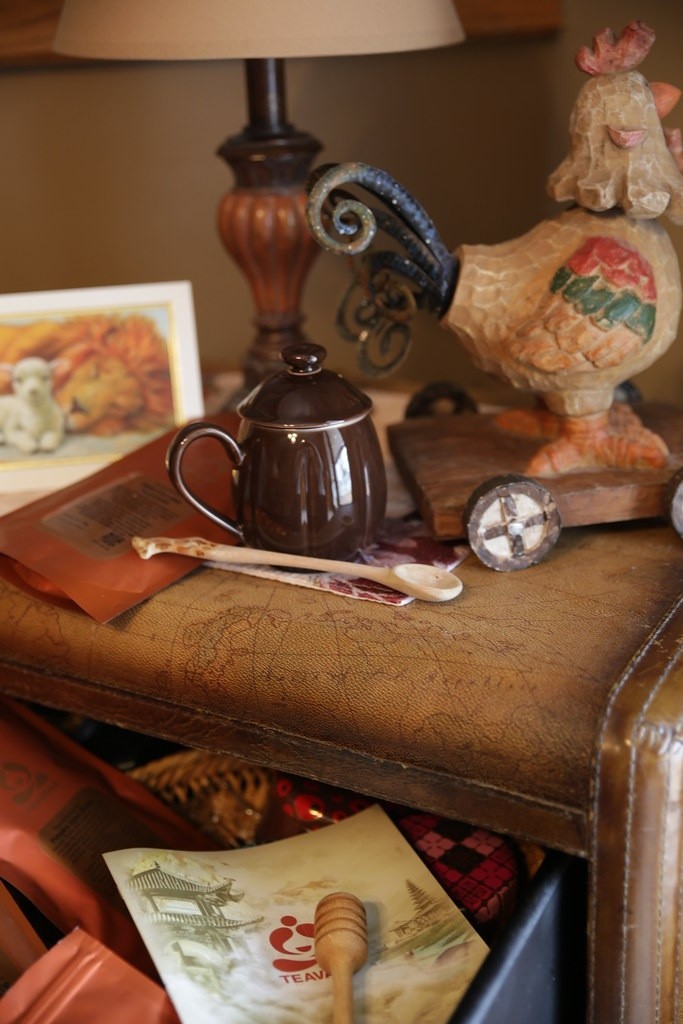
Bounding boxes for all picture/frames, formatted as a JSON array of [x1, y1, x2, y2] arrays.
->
[[0, 282, 205, 493]]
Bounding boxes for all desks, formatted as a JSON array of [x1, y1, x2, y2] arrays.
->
[[0, 529, 683, 1024]]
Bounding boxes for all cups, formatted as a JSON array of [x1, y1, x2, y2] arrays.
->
[[166, 342, 387, 560]]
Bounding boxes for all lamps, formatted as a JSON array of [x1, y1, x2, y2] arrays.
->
[[53, 0, 467, 390]]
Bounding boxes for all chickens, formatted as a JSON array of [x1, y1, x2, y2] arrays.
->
[[307, 19, 683, 481]]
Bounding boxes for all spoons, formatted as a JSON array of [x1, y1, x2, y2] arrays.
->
[[132, 536, 464, 602]]
